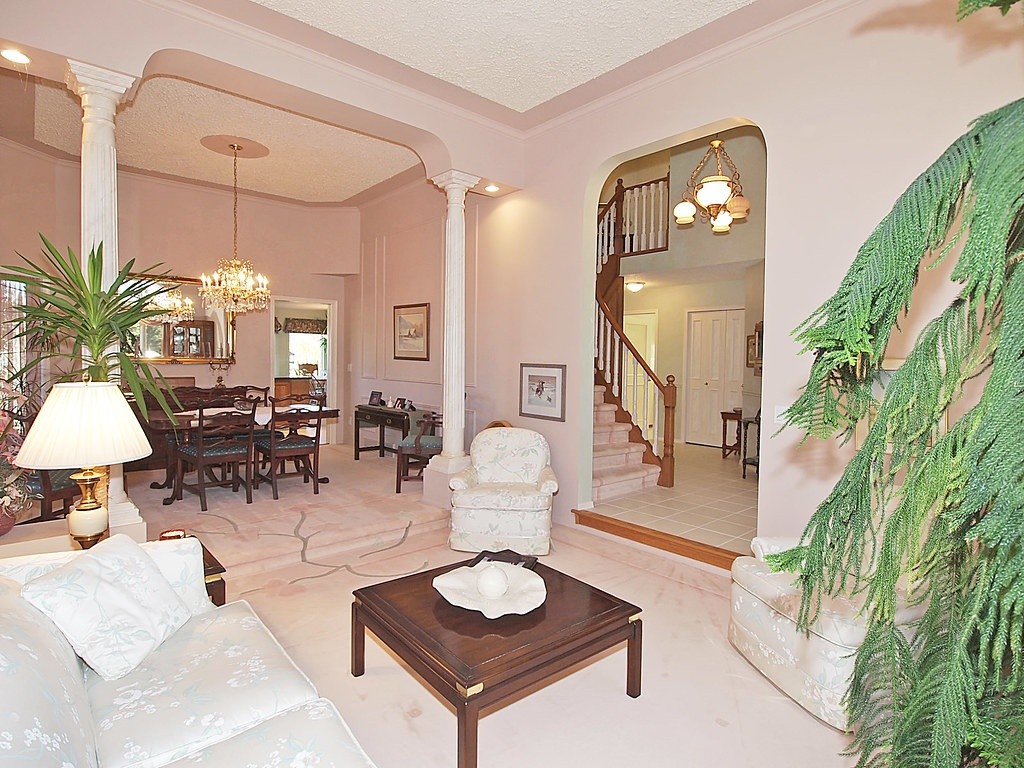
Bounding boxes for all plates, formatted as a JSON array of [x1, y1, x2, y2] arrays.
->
[[432, 561, 546, 619]]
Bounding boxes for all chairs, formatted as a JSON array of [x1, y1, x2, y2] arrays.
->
[[4, 404, 90, 521], [168, 397, 260, 511], [259, 391, 326, 500], [397, 394, 467, 493]]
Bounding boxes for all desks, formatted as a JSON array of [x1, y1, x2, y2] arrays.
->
[[721, 410, 742, 459], [139, 402, 341, 504], [352, 404, 433, 459]]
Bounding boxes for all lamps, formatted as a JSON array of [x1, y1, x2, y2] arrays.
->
[[131, 279, 194, 323], [198, 143, 270, 314], [13, 371, 156, 550], [675, 133, 750, 234]]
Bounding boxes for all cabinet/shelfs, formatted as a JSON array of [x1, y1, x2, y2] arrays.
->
[[275, 377, 313, 426], [161, 320, 213, 358]]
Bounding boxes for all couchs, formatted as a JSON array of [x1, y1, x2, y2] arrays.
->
[[0, 536, 376, 768], [730, 501, 939, 733], [447, 428, 556, 556]]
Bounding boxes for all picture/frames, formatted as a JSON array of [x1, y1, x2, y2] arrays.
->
[[854, 355, 948, 461], [392, 302, 431, 361], [746, 334, 756, 368], [520, 362, 566, 421]]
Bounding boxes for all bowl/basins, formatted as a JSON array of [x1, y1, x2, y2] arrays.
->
[[477, 565, 509, 600], [234, 401, 253, 410]]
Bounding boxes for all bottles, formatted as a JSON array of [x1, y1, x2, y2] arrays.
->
[[405, 402, 410, 410], [387, 396, 393, 408]]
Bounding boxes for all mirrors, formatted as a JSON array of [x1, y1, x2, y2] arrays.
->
[[116, 269, 236, 366]]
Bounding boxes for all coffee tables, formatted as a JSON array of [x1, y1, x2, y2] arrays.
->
[[351, 547, 643, 768]]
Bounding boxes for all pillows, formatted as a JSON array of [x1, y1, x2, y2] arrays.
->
[[18, 533, 191, 680]]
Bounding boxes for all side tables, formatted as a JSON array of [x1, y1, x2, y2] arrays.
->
[[145, 532, 227, 607]]
